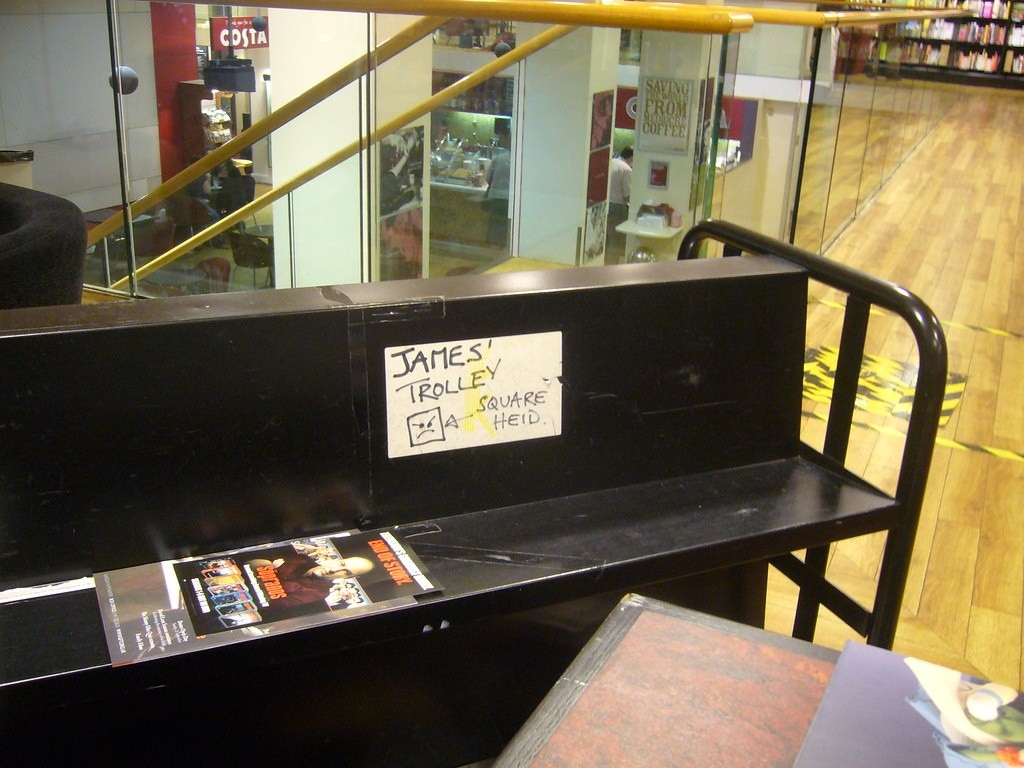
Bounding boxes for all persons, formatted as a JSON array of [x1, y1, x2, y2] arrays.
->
[[292, 539, 364, 610], [485, 148, 511, 250], [168, 156, 247, 252], [381, 129, 421, 209], [605, 143, 634, 265], [248, 553, 375, 608]]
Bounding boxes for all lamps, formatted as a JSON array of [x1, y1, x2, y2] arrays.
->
[[204, 5, 256, 97]]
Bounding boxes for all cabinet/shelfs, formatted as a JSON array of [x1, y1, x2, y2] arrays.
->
[[848, 0, 1024, 91]]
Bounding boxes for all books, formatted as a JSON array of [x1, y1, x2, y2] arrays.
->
[[967, 0, 1024, 22], [957, 21, 1024, 47], [884, 0, 968, 18], [838, 23, 879, 61], [885, 21, 953, 40], [842, 0, 883, 12], [879, 40, 950, 67], [952, 49, 1024, 75]]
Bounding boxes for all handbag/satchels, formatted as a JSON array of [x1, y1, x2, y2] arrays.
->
[[481, 181, 491, 213]]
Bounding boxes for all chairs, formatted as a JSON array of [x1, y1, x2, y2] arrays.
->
[[229, 232, 273, 289], [135, 217, 175, 257], [169, 192, 212, 249], [193, 256, 230, 293], [216, 176, 256, 232], [445, 18, 490, 47]]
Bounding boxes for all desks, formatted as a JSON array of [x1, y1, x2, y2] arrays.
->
[[83, 209, 158, 227], [495, 591, 841, 768], [244, 225, 273, 290], [145, 268, 207, 295], [615, 220, 683, 238]]
